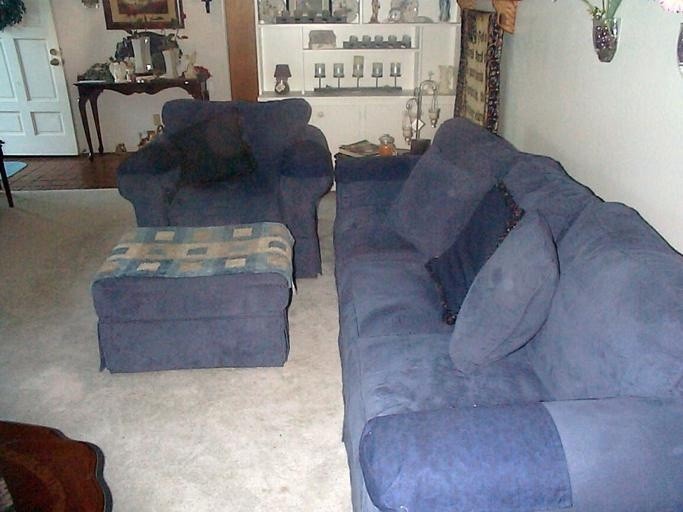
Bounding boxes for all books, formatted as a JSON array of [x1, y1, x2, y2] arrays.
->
[[337, 138, 381, 158]]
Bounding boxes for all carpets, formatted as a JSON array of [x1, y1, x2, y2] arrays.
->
[[1, 188, 352, 510], [0, 161, 27, 181]]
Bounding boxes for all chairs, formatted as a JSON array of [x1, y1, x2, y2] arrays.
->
[[0, 420, 113, 511], [117, 97, 333, 279]]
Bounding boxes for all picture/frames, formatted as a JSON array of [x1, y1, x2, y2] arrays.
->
[[102, 0, 185, 30]]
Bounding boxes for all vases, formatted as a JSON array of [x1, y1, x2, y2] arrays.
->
[[676, 23, 683, 73], [592, 18, 621, 63]]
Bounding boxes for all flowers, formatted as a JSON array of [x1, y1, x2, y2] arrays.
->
[[655, 0, 683, 15]]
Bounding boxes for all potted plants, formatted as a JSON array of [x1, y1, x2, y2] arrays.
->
[[157, 17, 188, 80], [109, 17, 153, 73]]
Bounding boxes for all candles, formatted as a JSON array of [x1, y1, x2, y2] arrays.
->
[[278, 8, 412, 78]]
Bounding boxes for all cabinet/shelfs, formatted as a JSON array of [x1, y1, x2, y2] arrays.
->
[[256, 0, 462, 96], [363, 96, 456, 150], [258, 97, 363, 192]]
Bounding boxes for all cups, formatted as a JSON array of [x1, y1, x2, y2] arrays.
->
[[378, 134, 398, 156]]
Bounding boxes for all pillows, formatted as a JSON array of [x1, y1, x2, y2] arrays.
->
[[448, 207, 560, 375], [424, 180, 525, 326], [170, 108, 257, 185], [390, 144, 494, 260]]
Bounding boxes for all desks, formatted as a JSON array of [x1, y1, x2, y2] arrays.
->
[[73, 65, 210, 162]]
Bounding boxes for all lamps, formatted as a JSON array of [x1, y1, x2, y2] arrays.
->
[[402, 80, 441, 140]]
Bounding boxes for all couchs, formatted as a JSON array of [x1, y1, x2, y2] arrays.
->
[[336, 118, 683, 511]]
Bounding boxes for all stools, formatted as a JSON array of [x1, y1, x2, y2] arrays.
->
[[91, 223, 293, 373]]
